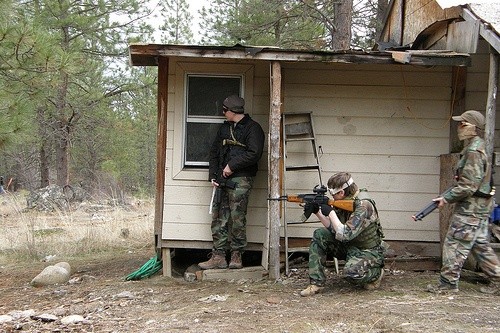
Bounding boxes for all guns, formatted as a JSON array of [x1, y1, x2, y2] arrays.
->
[[211, 142, 238, 218], [419, 184, 457, 222], [266, 187, 356, 223]]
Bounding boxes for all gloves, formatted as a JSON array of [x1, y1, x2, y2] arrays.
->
[[321, 204, 334, 216], [304, 203, 319, 214]]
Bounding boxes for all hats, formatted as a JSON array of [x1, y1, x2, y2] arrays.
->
[[223, 95, 245, 112], [452, 110, 485, 129]]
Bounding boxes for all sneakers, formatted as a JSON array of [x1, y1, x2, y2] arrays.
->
[[427, 279, 458, 292], [364, 268, 384, 289], [300, 285, 325, 296], [229, 250, 243, 269], [198, 253, 228, 269], [480, 278, 500, 293]]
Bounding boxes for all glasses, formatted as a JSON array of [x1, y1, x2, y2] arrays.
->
[[223, 108, 231, 112], [458, 122, 476, 127]]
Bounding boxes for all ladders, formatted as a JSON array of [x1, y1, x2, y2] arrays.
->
[[282, 111, 339, 275]]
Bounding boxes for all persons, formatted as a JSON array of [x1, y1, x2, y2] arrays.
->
[[198, 94, 266, 268], [301, 171, 385, 297], [424, 109, 500, 295]]
